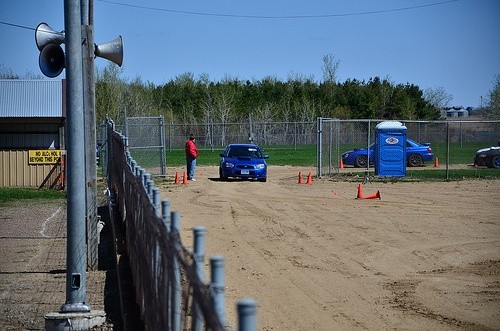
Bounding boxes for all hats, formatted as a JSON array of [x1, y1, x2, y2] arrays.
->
[[190, 134, 195, 140]]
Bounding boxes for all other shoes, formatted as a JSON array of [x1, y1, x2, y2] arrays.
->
[[188, 177, 196, 182]]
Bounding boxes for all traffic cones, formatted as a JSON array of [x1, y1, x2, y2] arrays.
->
[[354, 184, 364, 200], [305, 171, 313, 185], [338, 158, 344, 169], [296, 172, 304, 184], [364, 190, 381, 200], [433, 157, 439, 169], [173, 171, 181, 185], [181, 171, 190, 184]]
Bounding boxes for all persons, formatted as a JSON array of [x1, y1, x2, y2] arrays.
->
[[185, 135, 199, 181]]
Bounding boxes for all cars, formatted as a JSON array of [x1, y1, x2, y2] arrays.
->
[[474, 147, 500, 169], [218, 143, 270, 182], [341, 137, 435, 168]]
